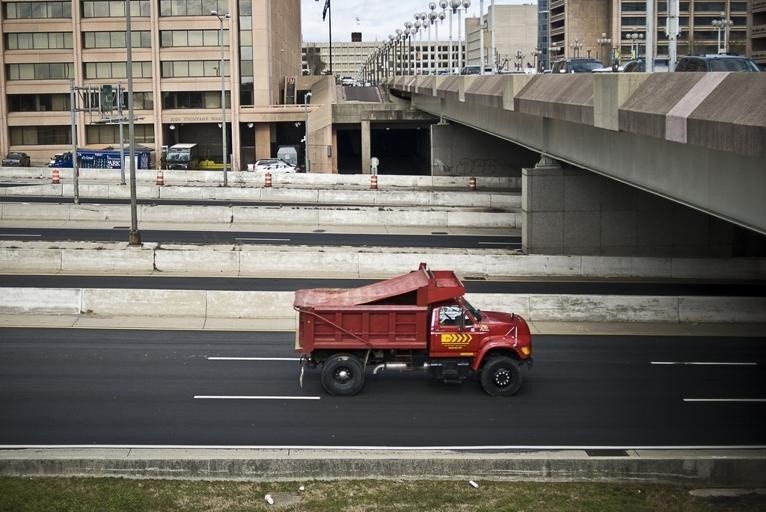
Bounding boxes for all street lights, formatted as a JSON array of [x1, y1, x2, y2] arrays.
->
[[355, 1, 471, 88], [501, 10, 735, 73], [211, 9, 232, 186], [304, 91, 312, 173]]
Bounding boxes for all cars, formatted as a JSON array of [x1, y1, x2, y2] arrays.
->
[[340, 76, 354, 86], [245, 158, 301, 174], [524, 52, 760, 73], [2, 151, 30, 166], [440, 63, 496, 75]]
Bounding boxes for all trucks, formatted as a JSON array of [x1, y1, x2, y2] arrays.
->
[[51, 148, 151, 169], [164, 141, 199, 168], [293, 262, 534, 396]]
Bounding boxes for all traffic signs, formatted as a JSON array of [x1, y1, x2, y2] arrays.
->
[[103, 84, 113, 112]]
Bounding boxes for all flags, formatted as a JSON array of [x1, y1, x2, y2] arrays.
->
[[321, 0, 329, 21]]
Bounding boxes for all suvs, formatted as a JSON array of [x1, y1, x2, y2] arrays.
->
[[48, 152, 64, 166]]
